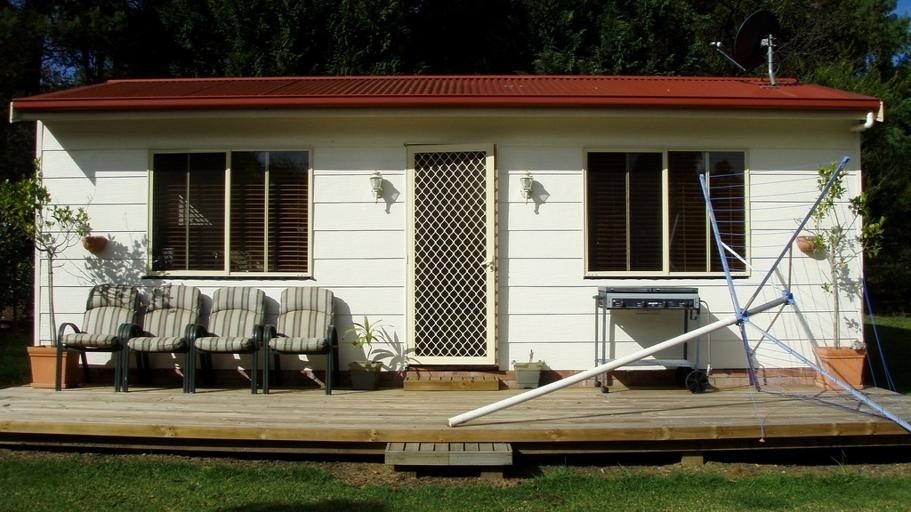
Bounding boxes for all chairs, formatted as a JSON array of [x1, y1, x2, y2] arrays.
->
[[55, 283, 141, 393], [121, 284, 204, 394], [190, 285, 266, 394], [262, 285, 334, 394]]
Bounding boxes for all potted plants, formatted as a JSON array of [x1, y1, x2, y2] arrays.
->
[[341, 312, 385, 390], [511, 349, 547, 389], [0, 157, 91, 389], [792, 160, 888, 392]]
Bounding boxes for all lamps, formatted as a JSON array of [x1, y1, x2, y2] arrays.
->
[[519, 169, 534, 206], [369, 167, 383, 204]]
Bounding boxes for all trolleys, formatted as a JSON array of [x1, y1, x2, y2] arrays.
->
[[591, 283, 711, 395]]
